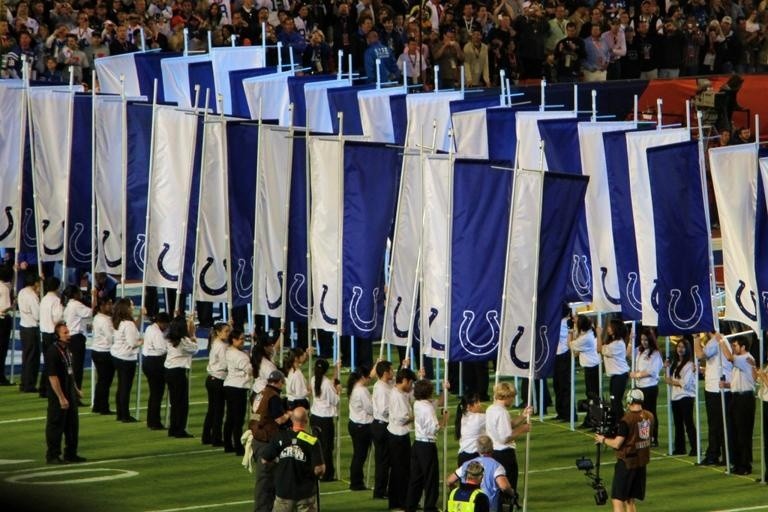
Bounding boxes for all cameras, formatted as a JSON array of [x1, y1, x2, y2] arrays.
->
[[564, 39, 572, 47], [446, 41, 456, 47]]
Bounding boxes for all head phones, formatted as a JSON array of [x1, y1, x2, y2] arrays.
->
[[626, 388, 633, 405]]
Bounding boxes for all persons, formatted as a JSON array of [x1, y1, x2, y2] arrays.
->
[[0, 0, 767, 97], [716, 75, 749, 129], [1, 267, 766, 511]]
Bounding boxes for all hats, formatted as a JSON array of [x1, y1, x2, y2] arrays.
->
[[270, 371, 286, 385]]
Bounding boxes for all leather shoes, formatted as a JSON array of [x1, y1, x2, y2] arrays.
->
[[78, 399, 89, 407], [0, 377, 48, 399], [64, 454, 86, 462], [321, 476, 370, 491], [202, 436, 245, 455], [46, 456, 67, 463], [91, 404, 193, 438]]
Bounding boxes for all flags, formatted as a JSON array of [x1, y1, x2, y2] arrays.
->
[[0, 43, 767, 380]]
